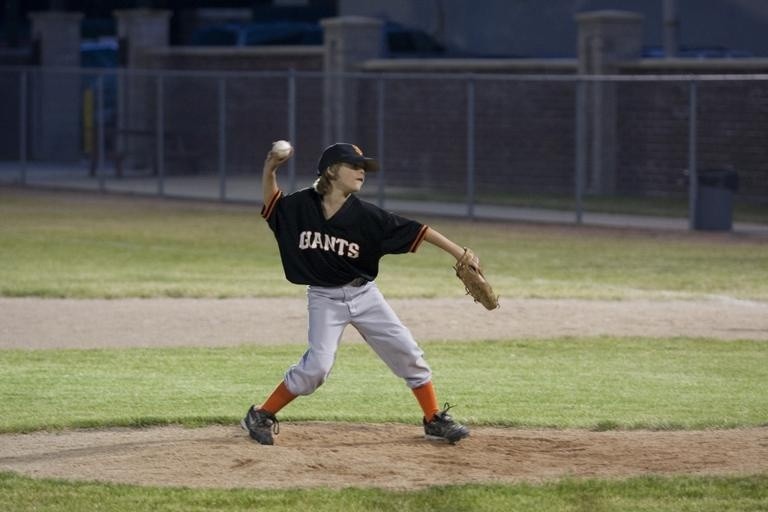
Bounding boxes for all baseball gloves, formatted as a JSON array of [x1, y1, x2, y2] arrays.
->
[[453, 246, 498, 311]]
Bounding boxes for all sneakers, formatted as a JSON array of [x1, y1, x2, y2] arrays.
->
[[240, 404, 276, 444], [423, 411, 471, 443]]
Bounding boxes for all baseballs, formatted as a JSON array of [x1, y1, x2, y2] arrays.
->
[[271, 140, 291, 161]]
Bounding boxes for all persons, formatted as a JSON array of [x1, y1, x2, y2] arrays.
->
[[239, 142, 480, 445]]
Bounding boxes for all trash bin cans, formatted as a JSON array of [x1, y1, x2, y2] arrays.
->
[[696, 167, 739, 230]]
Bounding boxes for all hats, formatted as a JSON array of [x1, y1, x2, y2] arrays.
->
[[317, 143, 380, 174]]
